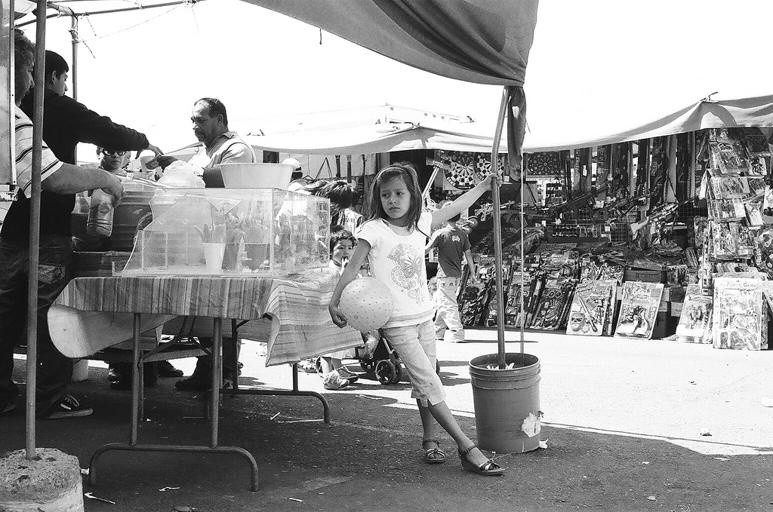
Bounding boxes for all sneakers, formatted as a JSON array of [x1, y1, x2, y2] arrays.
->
[[0, 359, 235, 418], [323, 367, 359, 388]]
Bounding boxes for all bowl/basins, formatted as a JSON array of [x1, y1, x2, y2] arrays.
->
[[215, 162, 296, 188]]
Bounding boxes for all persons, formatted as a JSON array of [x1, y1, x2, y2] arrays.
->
[[0, 51, 164, 424], [80, 145, 183, 390], [282, 159, 364, 229], [0, 24, 125, 238], [155, 96, 258, 392], [318, 230, 361, 389], [425, 200, 476, 342], [120, 151, 131, 170], [328, 162, 505, 476]]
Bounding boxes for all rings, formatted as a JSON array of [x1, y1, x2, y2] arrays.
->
[[121, 186, 124, 194]]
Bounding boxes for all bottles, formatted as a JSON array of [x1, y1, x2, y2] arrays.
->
[[82, 190, 113, 239]]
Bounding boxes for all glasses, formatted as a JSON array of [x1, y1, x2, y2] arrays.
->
[[100, 149, 128, 156]]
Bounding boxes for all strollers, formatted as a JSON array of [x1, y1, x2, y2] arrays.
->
[[315, 261, 440, 384]]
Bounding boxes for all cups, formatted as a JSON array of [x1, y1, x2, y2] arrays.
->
[[202, 241, 226, 271], [140, 155, 157, 176]]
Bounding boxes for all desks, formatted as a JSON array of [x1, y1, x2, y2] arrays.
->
[[74, 271, 349, 492]]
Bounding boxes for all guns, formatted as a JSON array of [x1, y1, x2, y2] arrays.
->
[[507, 182, 695, 256]]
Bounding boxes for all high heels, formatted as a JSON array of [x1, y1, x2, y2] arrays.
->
[[424, 438, 445, 464], [460, 446, 505, 475]]
